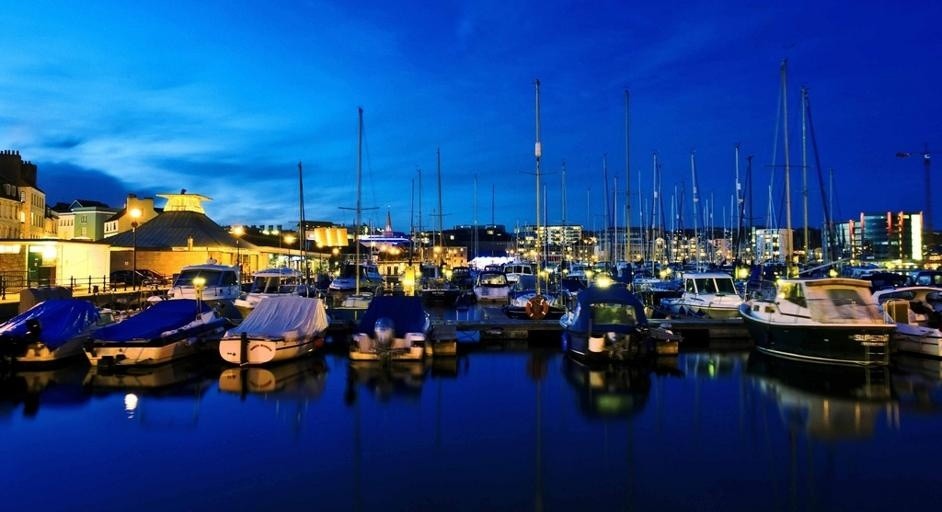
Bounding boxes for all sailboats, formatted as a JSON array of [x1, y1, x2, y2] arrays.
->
[[315, 56, 942, 380], [244, 159, 313, 305]]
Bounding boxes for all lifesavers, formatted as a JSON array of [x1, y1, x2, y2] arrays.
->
[[526, 297, 548, 319]]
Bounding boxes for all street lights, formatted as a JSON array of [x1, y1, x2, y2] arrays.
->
[[131, 208, 141, 291], [229, 222, 245, 267]]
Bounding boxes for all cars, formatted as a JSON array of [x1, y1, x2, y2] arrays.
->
[[110, 268, 166, 285]]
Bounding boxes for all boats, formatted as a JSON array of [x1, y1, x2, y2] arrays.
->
[[80, 301, 245, 370], [0, 297, 102, 365], [215, 356, 330, 394], [349, 359, 424, 397], [77, 363, 202, 388], [10, 366, 72, 396], [554, 349, 656, 416], [165, 261, 246, 305], [739, 355, 896, 439], [213, 289, 333, 366]]
